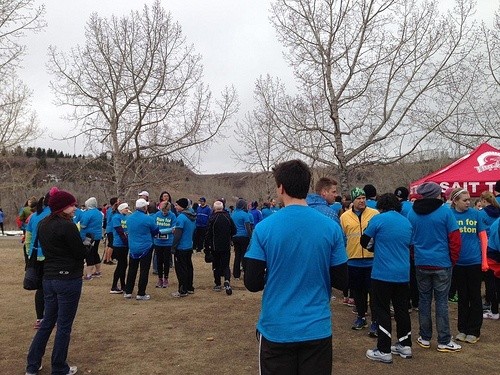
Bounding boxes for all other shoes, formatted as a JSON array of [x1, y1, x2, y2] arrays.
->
[[34, 318, 43, 329], [66, 365, 77, 375], [26, 365, 43, 375]]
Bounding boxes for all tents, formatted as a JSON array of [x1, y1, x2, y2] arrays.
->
[[408, 142, 500, 202]]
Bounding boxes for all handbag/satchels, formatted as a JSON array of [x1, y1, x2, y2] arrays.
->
[[204, 247, 213, 263], [22, 259, 45, 290]]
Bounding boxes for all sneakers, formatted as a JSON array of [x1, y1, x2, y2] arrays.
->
[[350, 316, 366, 330], [417, 336, 430, 348], [455, 332, 468, 341], [343, 290, 500, 319], [437, 340, 461, 352], [368, 322, 378, 338], [464, 334, 481, 343], [390, 342, 412, 358], [82, 256, 245, 299], [366, 347, 393, 363]]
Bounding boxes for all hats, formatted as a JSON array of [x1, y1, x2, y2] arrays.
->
[[416, 181, 442, 199], [138, 191, 149, 196], [176, 198, 188, 209], [117, 202, 128, 210], [198, 197, 206, 202], [351, 187, 364, 202], [363, 184, 376, 197], [136, 198, 149, 208], [395, 186, 410, 199], [48, 187, 76, 214]]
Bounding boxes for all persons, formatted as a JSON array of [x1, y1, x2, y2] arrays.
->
[[0, 176, 500, 375], [242, 159, 348, 375]]
